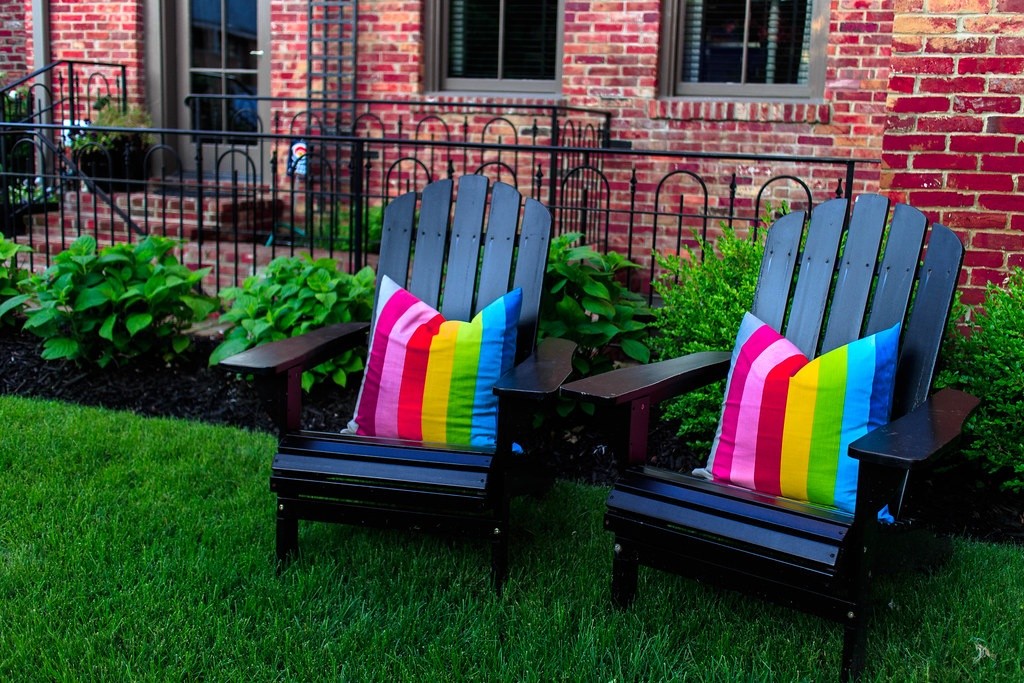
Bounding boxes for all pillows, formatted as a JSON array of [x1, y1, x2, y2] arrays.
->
[[691, 310, 902, 525], [340, 275, 524, 454]]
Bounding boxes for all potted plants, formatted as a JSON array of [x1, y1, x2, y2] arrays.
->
[[75, 100, 160, 194]]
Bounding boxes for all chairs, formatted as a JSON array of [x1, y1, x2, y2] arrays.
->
[[559, 192, 988, 683], [218, 173, 581, 596]]
